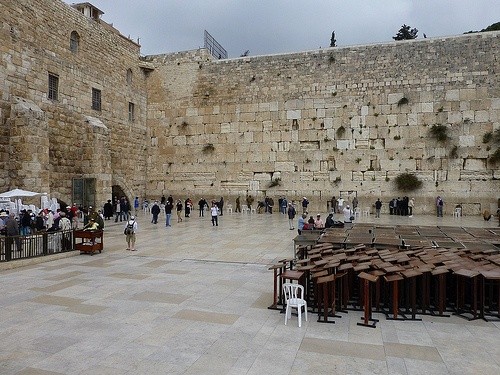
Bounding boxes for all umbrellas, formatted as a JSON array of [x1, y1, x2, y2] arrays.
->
[[0, 188, 49, 209]]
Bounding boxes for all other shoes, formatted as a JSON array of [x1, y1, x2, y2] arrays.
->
[[131, 249, 138, 251], [409, 214, 413, 217], [126, 248, 130, 250]]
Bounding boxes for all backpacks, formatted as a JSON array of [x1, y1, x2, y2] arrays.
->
[[126, 220, 135, 235], [439, 198, 443, 206]]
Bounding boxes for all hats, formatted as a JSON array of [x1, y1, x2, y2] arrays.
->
[[0, 212, 8, 217]]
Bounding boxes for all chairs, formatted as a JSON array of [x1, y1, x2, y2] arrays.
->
[[282, 283, 308, 328], [241, 204, 256, 214], [363, 207, 371, 216], [354, 207, 362, 219], [227, 204, 232, 213]]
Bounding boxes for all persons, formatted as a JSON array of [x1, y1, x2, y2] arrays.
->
[[142, 198, 149, 214], [133, 196, 139, 217], [161, 195, 323, 236], [150, 202, 160, 223], [123, 216, 137, 251], [374, 198, 382, 219], [0, 196, 131, 252], [387, 195, 416, 217], [324, 196, 358, 229], [436, 196, 443, 218]]
